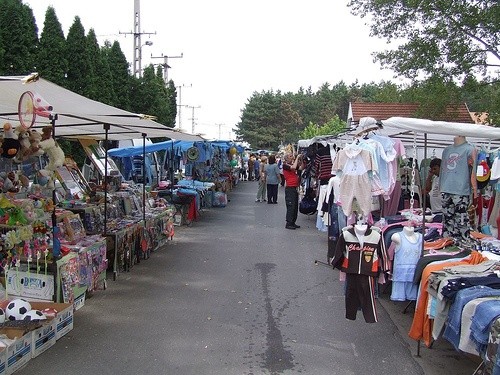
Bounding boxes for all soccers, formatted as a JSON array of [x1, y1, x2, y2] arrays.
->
[[0, 307, 5, 327], [21, 309, 47, 322], [5, 298, 32, 321]]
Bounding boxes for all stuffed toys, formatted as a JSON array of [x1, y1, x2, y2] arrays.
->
[[0, 123, 65, 192]]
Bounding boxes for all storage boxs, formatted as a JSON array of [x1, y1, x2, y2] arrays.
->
[[0, 263, 86, 375]]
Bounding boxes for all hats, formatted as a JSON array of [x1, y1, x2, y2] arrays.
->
[[355, 117, 378, 136]]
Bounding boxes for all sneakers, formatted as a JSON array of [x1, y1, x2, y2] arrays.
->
[[286, 223, 300, 229]]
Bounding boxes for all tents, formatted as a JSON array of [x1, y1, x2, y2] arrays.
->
[[297, 116, 500, 357], [107, 135, 252, 242], [0, 73, 211, 289]]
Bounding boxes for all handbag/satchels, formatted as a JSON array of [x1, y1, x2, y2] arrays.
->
[[299, 190, 318, 215]]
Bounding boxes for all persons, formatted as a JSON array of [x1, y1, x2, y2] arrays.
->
[[341, 224, 381, 323], [260, 153, 282, 205], [282, 152, 307, 230], [429, 158, 442, 210], [391, 226, 423, 300], [255, 156, 268, 202], [438, 135, 479, 246], [231, 148, 306, 193]]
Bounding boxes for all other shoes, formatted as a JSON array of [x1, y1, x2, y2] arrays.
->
[[268, 201, 273, 204], [256, 198, 260, 201], [260, 199, 264, 202], [273, 201, 277, 204]]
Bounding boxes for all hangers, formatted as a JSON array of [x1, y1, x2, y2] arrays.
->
[[356, 130, 436, 159]]
